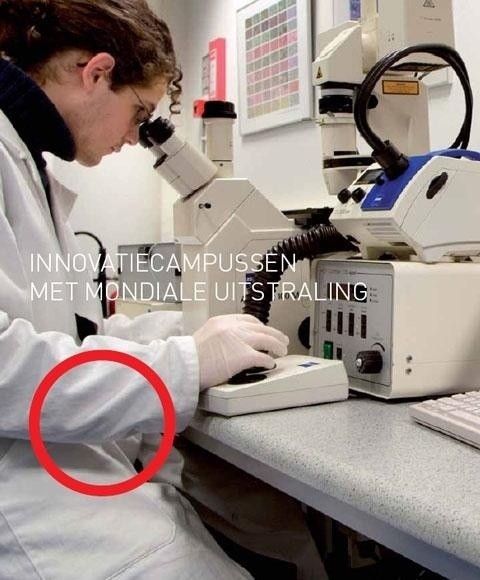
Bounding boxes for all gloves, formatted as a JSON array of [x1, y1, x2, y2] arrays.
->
[[192, 314, 289, 393]]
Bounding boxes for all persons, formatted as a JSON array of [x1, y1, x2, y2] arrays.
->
[[0, 0, 330, 579]]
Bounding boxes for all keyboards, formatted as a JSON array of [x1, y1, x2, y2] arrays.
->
[[409, 389, 480, 451]]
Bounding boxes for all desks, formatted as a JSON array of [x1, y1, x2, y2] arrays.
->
[[188, 400, 478, 578]]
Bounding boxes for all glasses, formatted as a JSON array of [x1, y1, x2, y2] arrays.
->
[[77, 62, 153, 126]]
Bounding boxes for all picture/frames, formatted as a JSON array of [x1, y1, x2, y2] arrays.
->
[[236, 1, 315, 137]]
